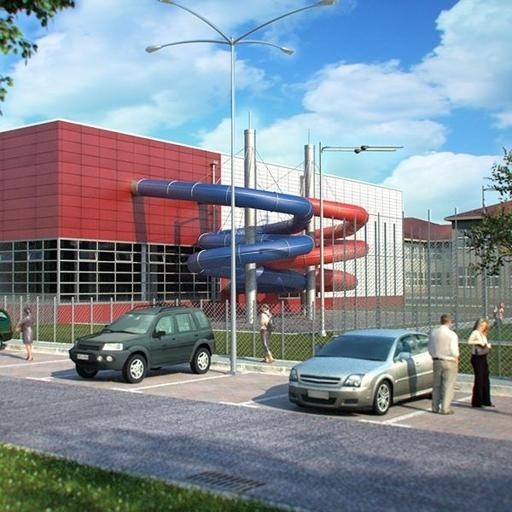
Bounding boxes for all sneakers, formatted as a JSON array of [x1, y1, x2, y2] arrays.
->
[[472, 403, 497, 409], [433, 406, 454, 415], [260, 355, 275, 364]]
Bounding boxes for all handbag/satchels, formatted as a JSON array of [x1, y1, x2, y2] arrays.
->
[[475, 345, 490, 356]]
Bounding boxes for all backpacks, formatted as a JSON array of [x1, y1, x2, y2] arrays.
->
[[262, 311, 276, 332]]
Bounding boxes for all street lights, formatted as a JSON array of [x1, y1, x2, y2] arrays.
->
[[145, 0, 336, 375], [319, 140, 366, 337]]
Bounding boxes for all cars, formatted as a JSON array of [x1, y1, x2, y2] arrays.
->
[[1, 308, 13, 350], [68, 303, 216, 384], [289, 328, 433, 415]]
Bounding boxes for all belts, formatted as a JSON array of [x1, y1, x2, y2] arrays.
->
[[432, 357, 444, 361]]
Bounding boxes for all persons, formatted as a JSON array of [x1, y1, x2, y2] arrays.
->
[[259, 302, 276, 364], [466, 317, 497, 410], [13, 305, 38, 362], [425, 311, 460, 415]]
